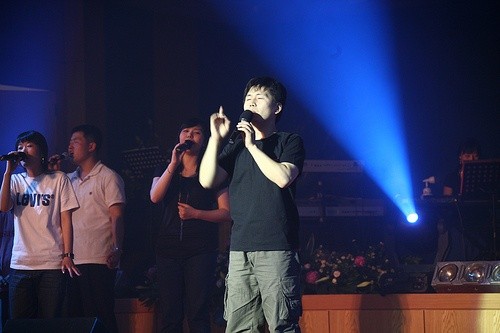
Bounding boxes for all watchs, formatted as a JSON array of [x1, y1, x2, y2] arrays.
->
[[63, 252, 74, 259]]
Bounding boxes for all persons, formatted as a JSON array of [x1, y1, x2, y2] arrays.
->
[[444, 143, 478, 197], [0, 131, 82, 319], [48, 124, 125, 333], [148, 119, 231, 333], [199, 75, 304, 333]]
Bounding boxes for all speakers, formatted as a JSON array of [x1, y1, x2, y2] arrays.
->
[[2, 317, 105, 333], [293, 197, 386, 257]]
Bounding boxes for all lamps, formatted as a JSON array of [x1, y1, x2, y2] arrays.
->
[[431, 260, 500, 285]]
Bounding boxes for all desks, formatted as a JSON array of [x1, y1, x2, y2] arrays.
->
[[115, 285, 500, 333]]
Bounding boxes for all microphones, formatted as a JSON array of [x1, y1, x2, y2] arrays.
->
[[1, 151, 27, 162], [49, 152, 69, 165], [229, 110, 253, 144], [176, 140, 193, 152]]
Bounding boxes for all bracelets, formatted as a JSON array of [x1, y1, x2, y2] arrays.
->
[[113, 248, 121, 254]]
